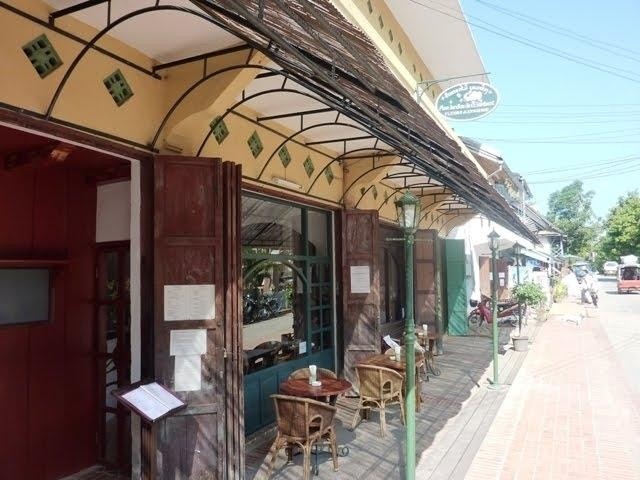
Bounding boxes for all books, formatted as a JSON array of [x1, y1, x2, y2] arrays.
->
[[121, 382, 185, 420]]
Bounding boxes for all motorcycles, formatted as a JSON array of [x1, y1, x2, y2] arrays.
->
[[243, 295, 283, 324], [581, 269, 598, 305], [468, 288, 527, 326], [618, 264, 640, 294]]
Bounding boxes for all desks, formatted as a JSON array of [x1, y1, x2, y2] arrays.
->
[[244, 347, 275, 374]]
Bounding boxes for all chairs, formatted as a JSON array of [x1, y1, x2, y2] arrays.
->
[[252, 330, 442, 479]]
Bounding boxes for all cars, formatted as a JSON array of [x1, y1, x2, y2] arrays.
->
[[573, 262, 591, 284], [603, 262, 618, 276]]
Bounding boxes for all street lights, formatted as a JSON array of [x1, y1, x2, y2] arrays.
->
[[394, 190, 422, 480], [512, 239, 522, 336], [487, 227, 501, 384]]
